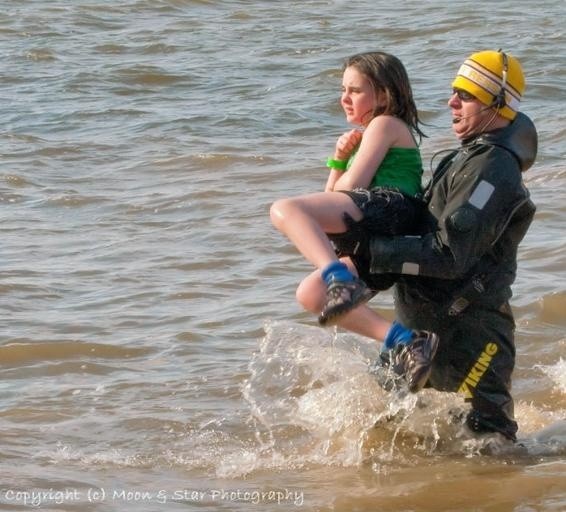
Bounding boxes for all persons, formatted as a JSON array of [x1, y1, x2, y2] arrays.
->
[[268, 52, 442, 393], [324, 51, 539, 445]]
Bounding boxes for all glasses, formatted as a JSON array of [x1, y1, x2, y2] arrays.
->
[[452, 85, 477, 102]]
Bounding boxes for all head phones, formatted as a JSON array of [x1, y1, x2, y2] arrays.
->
[[492, 49, 508, 109]]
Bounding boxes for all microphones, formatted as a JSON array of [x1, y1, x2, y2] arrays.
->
[[452, 100, 501, 124]]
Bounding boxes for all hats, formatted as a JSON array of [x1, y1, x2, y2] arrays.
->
[[450, 50, 526, 121]]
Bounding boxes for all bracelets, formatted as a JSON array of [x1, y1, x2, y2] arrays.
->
[[326, 158, 348, 169]]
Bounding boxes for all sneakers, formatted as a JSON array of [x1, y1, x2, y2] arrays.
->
[[396, 327, 441, 396], [317, 272, 377, 326]]
